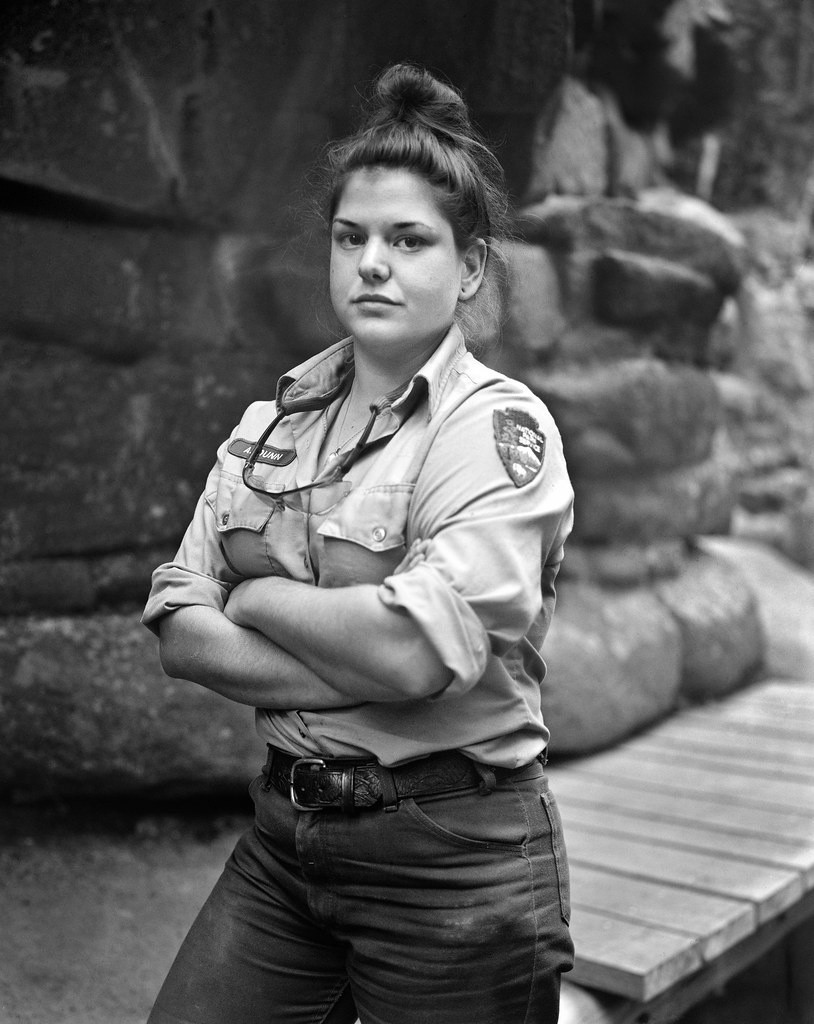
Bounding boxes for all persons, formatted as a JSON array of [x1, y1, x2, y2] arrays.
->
[[141, 63, 574, 1023]]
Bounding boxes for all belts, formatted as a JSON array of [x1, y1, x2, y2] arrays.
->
[[262, 742, 538, 812]]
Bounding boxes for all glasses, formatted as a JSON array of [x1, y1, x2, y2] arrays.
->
[[242, 404, 381, 516]]
